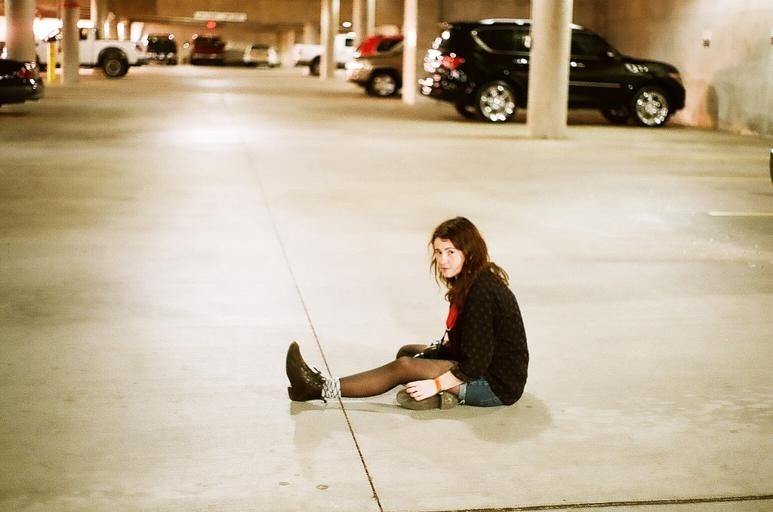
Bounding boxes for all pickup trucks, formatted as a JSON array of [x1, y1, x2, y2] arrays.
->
[[35, 27, 148, 77], [292, 32, 354, 76]]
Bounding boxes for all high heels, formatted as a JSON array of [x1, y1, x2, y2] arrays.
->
[[397, 388, 457, 410], [286, 341, 326, 401]]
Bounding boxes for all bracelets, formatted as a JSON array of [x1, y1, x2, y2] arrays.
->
[[434, 374, 443, 394]]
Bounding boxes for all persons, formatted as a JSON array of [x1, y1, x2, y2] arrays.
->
[[286, 215, 530, 411]]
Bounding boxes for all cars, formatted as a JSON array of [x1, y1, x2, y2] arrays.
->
[[345, 34, 404, 96], [147, 34, 280, 68], [418, 18, 685, 127], [0, 59, 43, 105]]
